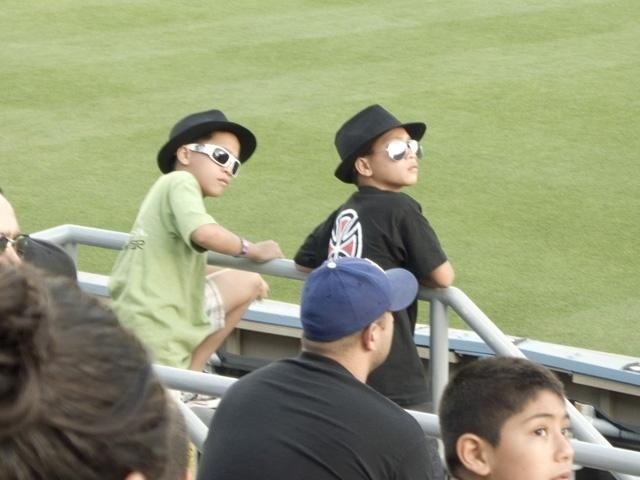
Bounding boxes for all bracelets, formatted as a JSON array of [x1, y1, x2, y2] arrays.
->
[[233, 236, 250, 258]]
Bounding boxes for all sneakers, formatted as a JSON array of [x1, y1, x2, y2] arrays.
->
[[181, 391, 219, 407]]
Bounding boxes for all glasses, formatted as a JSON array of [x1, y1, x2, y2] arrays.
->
[[0, 231, 31, 261], [361, 140, 426, 163], [184, 143, 242, 177]]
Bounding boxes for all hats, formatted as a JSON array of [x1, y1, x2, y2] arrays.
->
[[155, 109, 259, 177], [331, 103, 429, 184], [299, 258, 420, 343]]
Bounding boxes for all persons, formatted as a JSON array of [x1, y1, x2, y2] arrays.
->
[[437, 354, 575, 480], [294, 103, 455, 413], [1, 186, 27, 265], [103, 109, 285, 372], [195, 256, 447, 479], [0, 264, 192, 480]]
